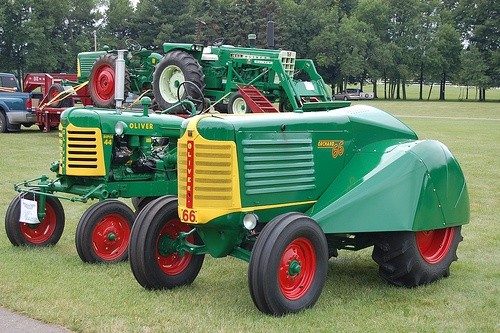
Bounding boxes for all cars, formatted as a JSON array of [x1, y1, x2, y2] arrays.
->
[[333, 90, 359, 101]]
